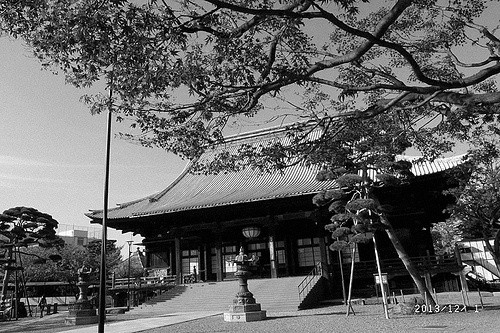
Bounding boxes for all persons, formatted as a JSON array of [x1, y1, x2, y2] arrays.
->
[[38, 295, 48, 318]]
